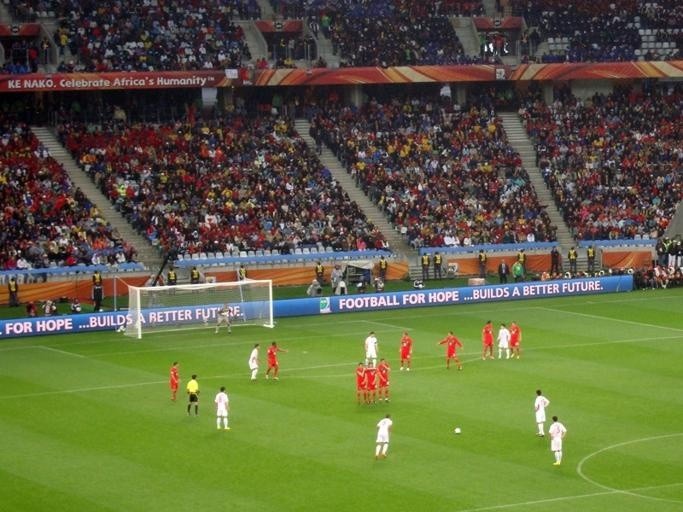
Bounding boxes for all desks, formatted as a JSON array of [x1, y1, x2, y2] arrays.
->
[[468, 277, 485, 286]]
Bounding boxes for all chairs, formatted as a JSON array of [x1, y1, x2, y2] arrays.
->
[[0, 262, 145, 284], [578, 234, 652, 248], [177, 247, 391, 269], [418, 241, 560, 255], [548, 38, 569, 50], [627, 3, 683, 60]]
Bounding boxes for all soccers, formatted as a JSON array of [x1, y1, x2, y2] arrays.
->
[[455, 427, 460, 434]]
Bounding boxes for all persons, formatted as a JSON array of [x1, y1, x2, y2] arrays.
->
[[549, 416, 567, 466], [1, 3, 683, 317], [249, 344, 260, 380], [496, 323, 512, 359], [214, 302, 234, 334], [398, 330, 412, 371], [375, 414, 393, 460], [438, 332, 463, 370], [356, 331, 391, 405], [214, 386, 231, 430], [265, 341, 288, 380], [169, 361, 180, 400], [508, 321, 522, 359], [534, 389, 550, 437], [482, 320, 496, 360], [185, 374, 200, 417]]
